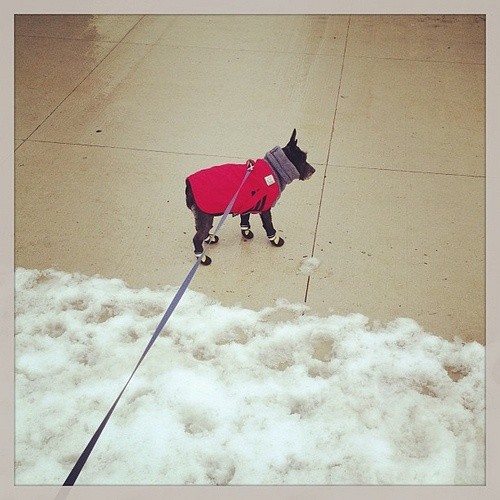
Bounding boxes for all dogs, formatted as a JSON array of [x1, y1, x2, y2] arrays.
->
[[184, 128, 317, 266]]
